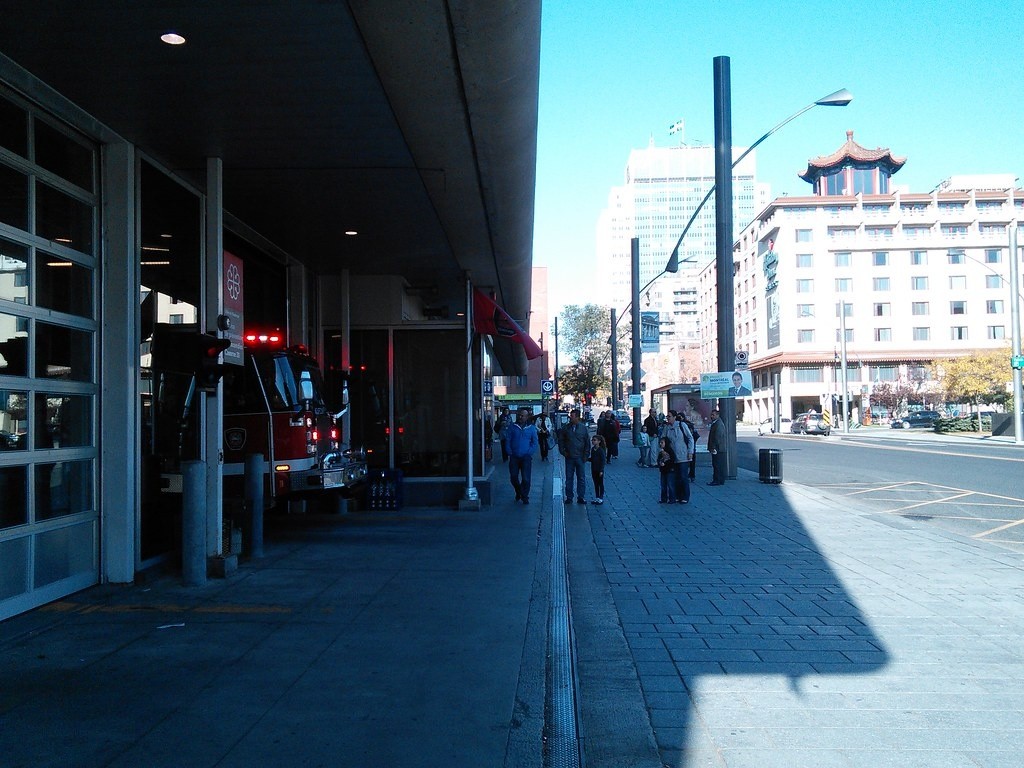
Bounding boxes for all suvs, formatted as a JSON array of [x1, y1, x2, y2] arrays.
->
[[789, 412, 830, 436], [890, 410, 942, 430]]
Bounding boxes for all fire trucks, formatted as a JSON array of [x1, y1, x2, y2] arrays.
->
[[138, 328, 367, 530]]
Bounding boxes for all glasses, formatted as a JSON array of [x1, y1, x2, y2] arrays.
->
[[666, 416, 671, 418], [591, 439, 598, 441], [642, 428, 647, 429], [653, 411, 656, 413], [607, 413, 610, 414]]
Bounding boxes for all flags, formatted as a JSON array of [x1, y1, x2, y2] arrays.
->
[[470, 283, 544, 361]]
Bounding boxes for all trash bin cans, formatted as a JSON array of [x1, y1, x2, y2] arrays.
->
[[759, 448, 783, 484]]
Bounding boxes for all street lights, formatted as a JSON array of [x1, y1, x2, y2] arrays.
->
[[665, 56, 855, 481], [607, 238, 697, 442]]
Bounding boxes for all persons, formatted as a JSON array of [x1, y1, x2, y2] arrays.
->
[[493, 407, 621, 505], [685, 399, 703, 422], [729, 372, 752, 396], [637, 407, 728, 504]]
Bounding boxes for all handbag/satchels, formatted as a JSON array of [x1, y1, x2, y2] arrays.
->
[[679, 422, 689, 445], [691, 427, 700, 442], [546, 429, 555, 450], [494, 415, 502, 433]]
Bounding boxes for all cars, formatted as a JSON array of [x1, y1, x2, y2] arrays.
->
[[968, 410, 999, 421], [612, 410, 631, 430], [758, 417, 790, 436]]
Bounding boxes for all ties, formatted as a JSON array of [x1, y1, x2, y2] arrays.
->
[[735, 389, 738, 395]]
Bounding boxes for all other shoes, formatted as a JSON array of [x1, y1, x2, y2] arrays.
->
[[667, 499, 688, 504], [607, 461, 611, 464], [542, 458, 548, 461], [638, 461, 659, 468], [577, 499, 587, 504], [659, 500, 667, 503], [591, 498, 603, 505], [612, 456, 618, 459], [519, 494, 530, 504], [691, 478, 695, 483], [564, 500, 573, 504], [707, 482, 724, 486], [503, 459, 507, 463], [516, 492, 520, 501]]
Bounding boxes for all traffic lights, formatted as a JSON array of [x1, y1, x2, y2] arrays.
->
[[587, 391, 592, 406]]
